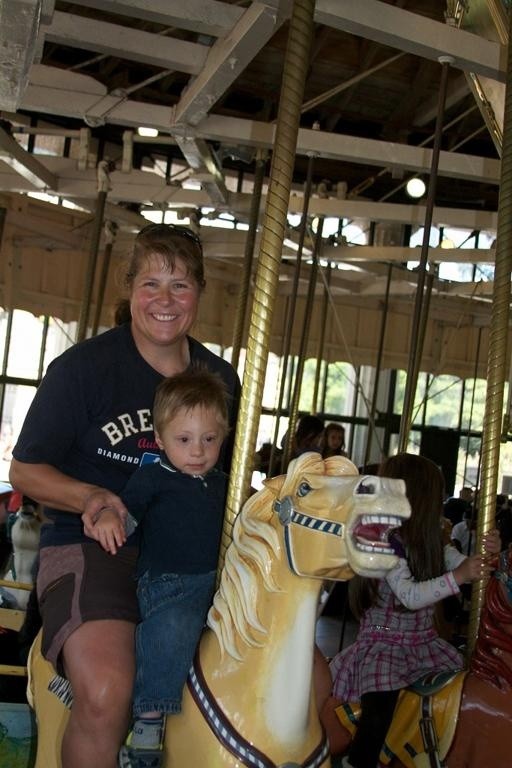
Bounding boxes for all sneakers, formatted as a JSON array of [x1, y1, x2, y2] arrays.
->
[[127, 729, 165, 768]]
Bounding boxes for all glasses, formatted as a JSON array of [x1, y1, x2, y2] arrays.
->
[[133, 224, 203, 252]]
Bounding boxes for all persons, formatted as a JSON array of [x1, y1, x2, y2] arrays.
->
[[256, 414, 348, 478], [443, 488, 512, 558], [0, 223, 333, 768], [326, 454, 499, 768]]
